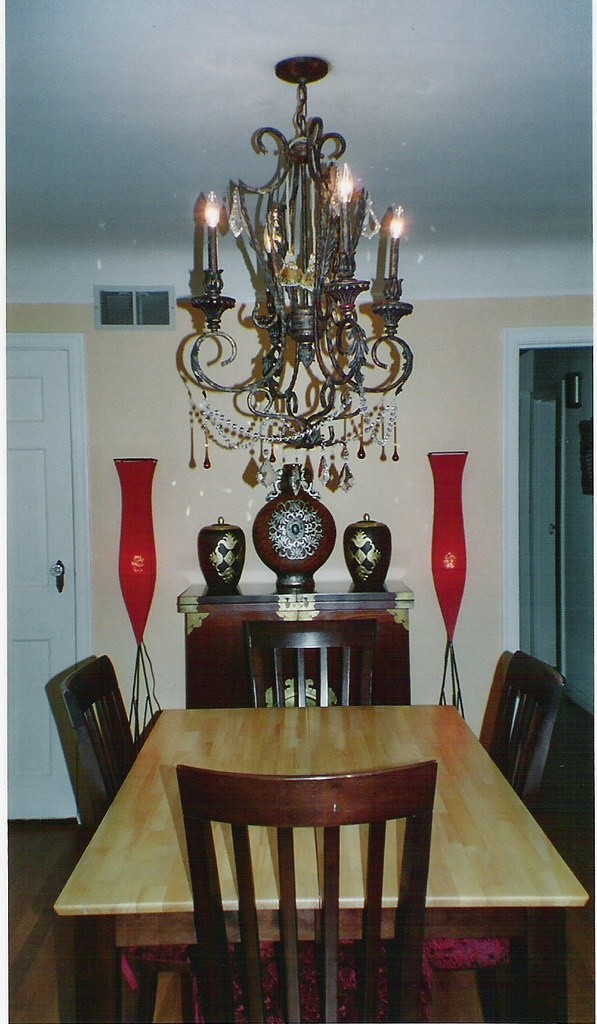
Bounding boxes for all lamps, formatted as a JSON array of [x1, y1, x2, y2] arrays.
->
[[188, 55, 414, 497]]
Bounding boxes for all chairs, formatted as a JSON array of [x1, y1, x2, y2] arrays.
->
[[241, 618, 379, 708], [61, 654, 198, 1023], [176, 758, 440, 1024], [426, 650, 566, 1024]]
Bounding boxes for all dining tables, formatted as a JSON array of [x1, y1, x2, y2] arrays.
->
[[53, 704, 591, 1024]]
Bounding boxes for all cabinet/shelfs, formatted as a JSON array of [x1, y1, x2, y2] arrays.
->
[[176, 580, 417, 711]]
